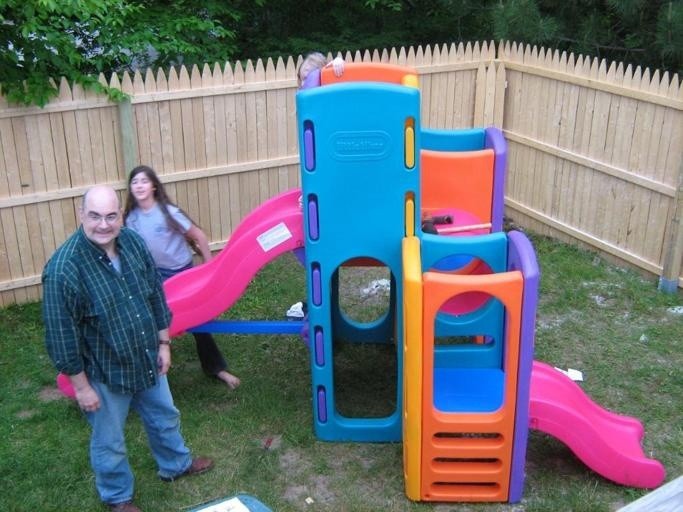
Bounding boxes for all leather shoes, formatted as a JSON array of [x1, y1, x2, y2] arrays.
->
[[110, 502, 144, 512], [159, 454, 215, 482]]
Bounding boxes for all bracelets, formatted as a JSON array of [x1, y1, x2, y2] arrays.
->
[[158, 339, 170, 345]]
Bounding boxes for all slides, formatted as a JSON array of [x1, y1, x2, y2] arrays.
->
[[58, 189, 304, 397], [526, 362, 664, 488]]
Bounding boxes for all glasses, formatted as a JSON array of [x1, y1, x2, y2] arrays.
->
[[81, 208, 121, 224]]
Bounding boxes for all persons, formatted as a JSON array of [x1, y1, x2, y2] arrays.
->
[[297, 52, 345, 210], [40, 183, 216, 510], [119, 164, 240, 390]]
